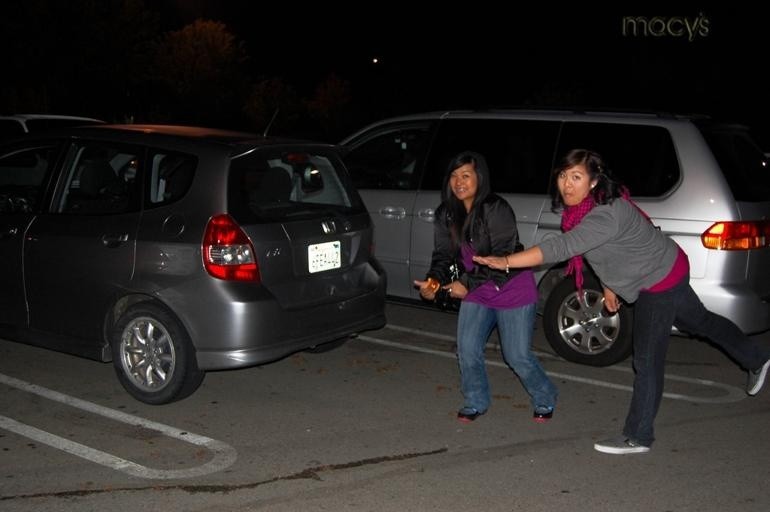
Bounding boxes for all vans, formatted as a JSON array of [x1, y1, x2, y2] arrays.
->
[[290, 105, 770, 367]]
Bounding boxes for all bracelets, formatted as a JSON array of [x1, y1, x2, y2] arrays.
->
[[505, 255, 509, 274]]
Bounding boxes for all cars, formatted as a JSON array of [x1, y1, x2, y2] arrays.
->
[[0, 123, 388, 406]]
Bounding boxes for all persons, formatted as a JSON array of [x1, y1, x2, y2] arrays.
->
[[471, 149, 770, 455], [413, 151, 559, 422]]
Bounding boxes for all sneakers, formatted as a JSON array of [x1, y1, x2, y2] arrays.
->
[[593, 434, 651, 456], [456, 406, 489, 424], [747, 355, 770, 396], [531, 404, 554, 424]]
